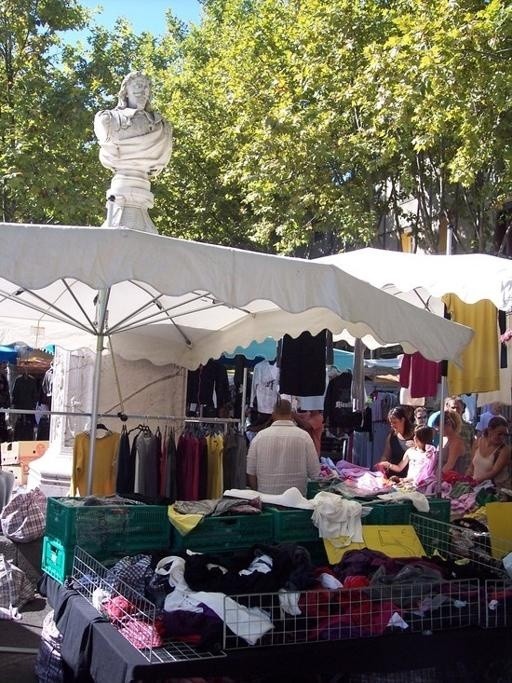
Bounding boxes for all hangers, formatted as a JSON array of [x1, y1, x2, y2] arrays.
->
[[96, 414, 246, 438]]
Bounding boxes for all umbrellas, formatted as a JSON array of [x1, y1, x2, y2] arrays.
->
[[324, 247, 512, 493], [0, 223, 475, 496]]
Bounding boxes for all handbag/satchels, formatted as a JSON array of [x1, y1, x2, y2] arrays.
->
[[0, 485, 47, 543], [34, 611, 67, 683], [0, 554, 35, 621]]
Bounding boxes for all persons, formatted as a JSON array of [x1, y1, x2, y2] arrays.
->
[[295, 410, 323, 457], [246, 399, 320, 498], [376, 396, 512, 490], [94, 70, 174, 172]]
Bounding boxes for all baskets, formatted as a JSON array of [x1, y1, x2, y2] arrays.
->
[[41, 484, 450, 583]]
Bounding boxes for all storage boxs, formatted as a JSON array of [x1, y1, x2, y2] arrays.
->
[[0, 439, 49, 465], [0, 463, 28, 489]]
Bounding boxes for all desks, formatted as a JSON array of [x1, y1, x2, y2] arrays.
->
[[35, 546, 512, 682]]
